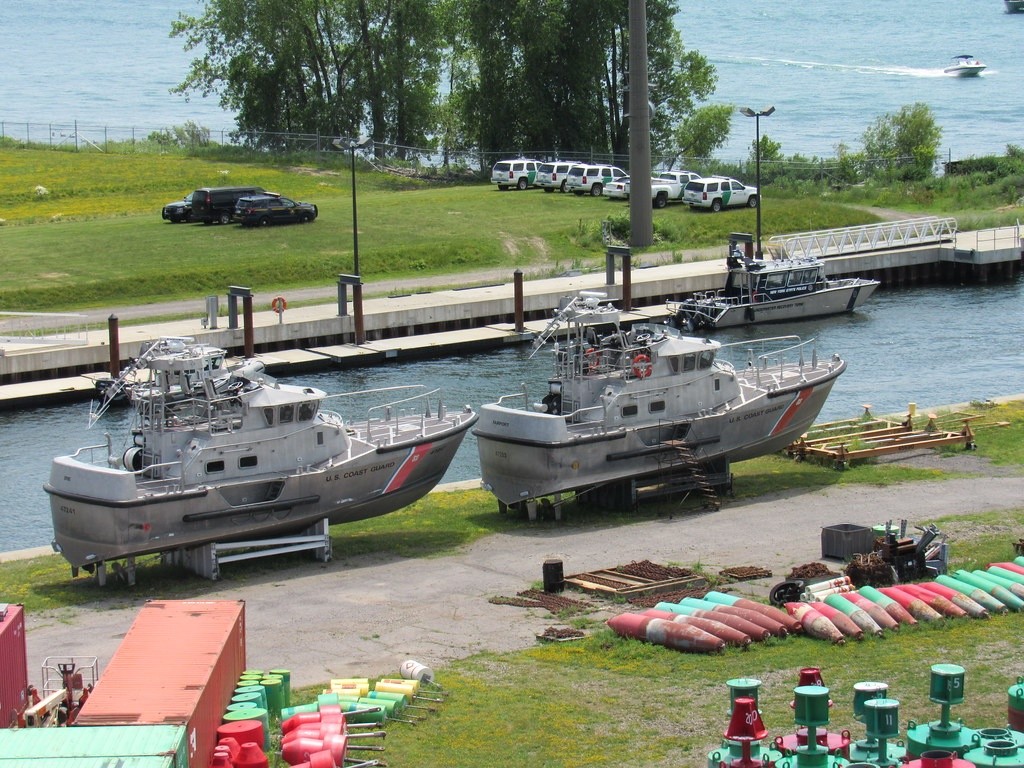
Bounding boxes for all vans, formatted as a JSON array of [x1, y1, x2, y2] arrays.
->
[[682, 175, 762, 212], [162, 187, 267, 225], [491, 156, 702, 209]]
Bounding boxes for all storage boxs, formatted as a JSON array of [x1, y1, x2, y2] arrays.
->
[[70, 598, 247, 768], [0, 723, 190, 768], [0, 603, 29, 730], [818, 523, 873, 561]]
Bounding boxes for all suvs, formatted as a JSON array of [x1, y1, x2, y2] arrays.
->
[[234, 192, 318, 227]]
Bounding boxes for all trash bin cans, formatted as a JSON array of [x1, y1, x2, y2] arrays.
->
[[543, 559, 565, 593]]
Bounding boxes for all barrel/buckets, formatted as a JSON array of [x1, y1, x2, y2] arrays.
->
[[543, 559, 565, 593]]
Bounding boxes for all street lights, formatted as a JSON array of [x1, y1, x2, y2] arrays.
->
[[740, 105, 776, 260], [332, 138, 374, 276]]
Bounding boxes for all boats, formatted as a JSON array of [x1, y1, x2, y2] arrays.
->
[[944, 55, 987, 77], [663, 232, 882, 333], [43, 336, 480, 587], [471, 291, 847, 520]]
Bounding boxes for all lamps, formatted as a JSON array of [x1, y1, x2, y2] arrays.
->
[[107, 457, 123, 467]]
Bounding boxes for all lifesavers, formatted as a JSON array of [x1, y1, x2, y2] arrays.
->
[[585, 348, 601, 369], [271, 297, 288, 314], [632, 354, 652, 377]]
